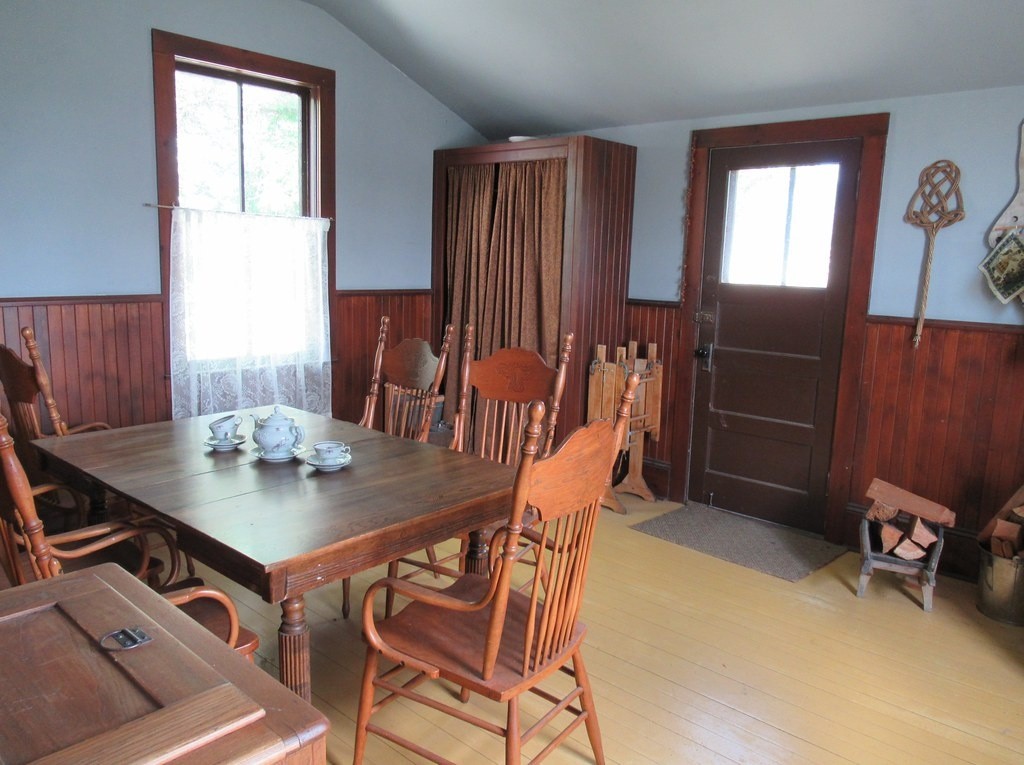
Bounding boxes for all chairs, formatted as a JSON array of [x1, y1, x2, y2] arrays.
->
[[0, 505, 165, 592], [856, 518, 945, 612], [0, 413, 261, 665], [0, 327, 141, 520], [342, 315, 455, 622], [351, 369, 642, 765], [384, 323, 574, 620]]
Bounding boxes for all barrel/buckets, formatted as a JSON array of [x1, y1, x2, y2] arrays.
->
[[976, 540, 1024, 625]]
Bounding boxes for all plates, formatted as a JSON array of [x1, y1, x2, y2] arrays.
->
[[508, 136, 538, 142], [204, 435, 247, 451], [305, 453, 351, 471], [248, 445, 307, 462]]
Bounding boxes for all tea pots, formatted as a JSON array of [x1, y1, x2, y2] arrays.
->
[[249, 405, 304, 454]]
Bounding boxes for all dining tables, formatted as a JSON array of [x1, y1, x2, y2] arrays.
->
[[26, 401, 520, 705]]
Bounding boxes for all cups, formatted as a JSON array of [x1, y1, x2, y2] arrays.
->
[[313, 441, 351, 464], [208, 415, 243, 442]]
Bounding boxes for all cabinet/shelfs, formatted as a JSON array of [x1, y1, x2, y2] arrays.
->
[[586, 341, 663, 514], [0, 561, 332, 765], [431, 134, 637, 487]]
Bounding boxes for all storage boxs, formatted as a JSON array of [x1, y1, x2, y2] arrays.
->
[[976, 540, 1024, 627]]
